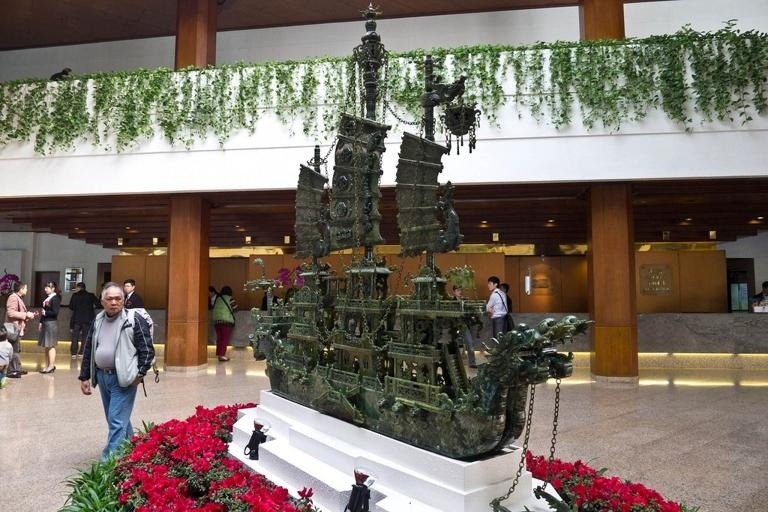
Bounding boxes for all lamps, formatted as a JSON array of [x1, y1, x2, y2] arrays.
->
[[243, 417, 273, 460], [343, 467, 377, 512]]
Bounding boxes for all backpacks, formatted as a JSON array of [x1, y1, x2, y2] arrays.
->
[[95, 308, 154, 345]]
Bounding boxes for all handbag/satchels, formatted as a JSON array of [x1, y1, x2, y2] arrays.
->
[[505, 312, 515, 333], [3, 319, 20, 343]]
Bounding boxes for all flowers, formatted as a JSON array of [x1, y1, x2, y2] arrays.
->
[[0, 273, 20, 296], [271, 266, 304, 289]]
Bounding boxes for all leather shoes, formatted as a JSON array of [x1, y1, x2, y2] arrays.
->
[[218, 356, 230, 362], [469, 363, 479, 369]]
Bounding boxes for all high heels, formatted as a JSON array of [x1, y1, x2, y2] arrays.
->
[[40, 366, 56, 373]]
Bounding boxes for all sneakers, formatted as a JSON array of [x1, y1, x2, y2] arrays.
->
[[7, 370, 27, 378], [71, 355, 83, 359]]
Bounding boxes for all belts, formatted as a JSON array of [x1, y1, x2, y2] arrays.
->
[[96, 366, 117, 374]]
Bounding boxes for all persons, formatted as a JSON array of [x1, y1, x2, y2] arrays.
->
[[78, 282, 156, 462], [484, 277, 507, 337], [212, 286, 239, 361], [208, 287, 219, 310], [68, 283, 103, 359], [124, 279, 143, 308], [262, 292, 279, 310], [753, 281, 768, 306], [499, 283, 512, 331], [5, 283, 35, 378], [453, 285, 475, 368], [0, 326, 13, 389], [38, 281, 62, 373]]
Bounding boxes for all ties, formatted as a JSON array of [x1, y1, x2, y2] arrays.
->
[[124, 295, 130, 307]]
